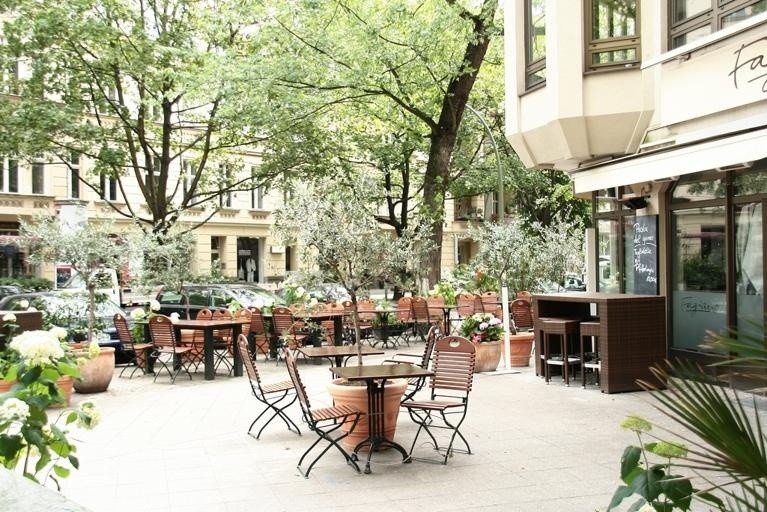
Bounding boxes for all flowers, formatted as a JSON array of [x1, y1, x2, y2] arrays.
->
[[10, 329, 82, 381]]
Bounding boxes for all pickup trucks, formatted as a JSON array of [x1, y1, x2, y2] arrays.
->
[[61, 270, 156, 316]]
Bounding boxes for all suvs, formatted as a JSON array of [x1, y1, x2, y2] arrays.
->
[[231, 286, 281, 306], [564, 272, 583, 289], [582, 255, 616, 284], [156, 285, 246, 317]]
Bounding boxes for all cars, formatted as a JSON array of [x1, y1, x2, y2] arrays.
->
[[535, 277, 566, 293], [1, 285, 32, 295], [1, 294, 138, 364], [307, 282, 350, 298]]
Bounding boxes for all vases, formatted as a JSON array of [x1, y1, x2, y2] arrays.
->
[[48, 375, 73, 407], [71, 347, 115, 392]]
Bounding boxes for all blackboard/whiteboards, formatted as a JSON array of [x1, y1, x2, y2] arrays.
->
[[625, 214, 659, 297]]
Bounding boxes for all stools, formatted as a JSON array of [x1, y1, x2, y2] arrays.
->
[[538, 310, 599, 388]]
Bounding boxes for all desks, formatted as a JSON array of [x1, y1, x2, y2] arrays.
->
[[533, 293, 667, 394]]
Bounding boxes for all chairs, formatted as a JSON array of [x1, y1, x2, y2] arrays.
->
[[237, 325, 476, 478]]
